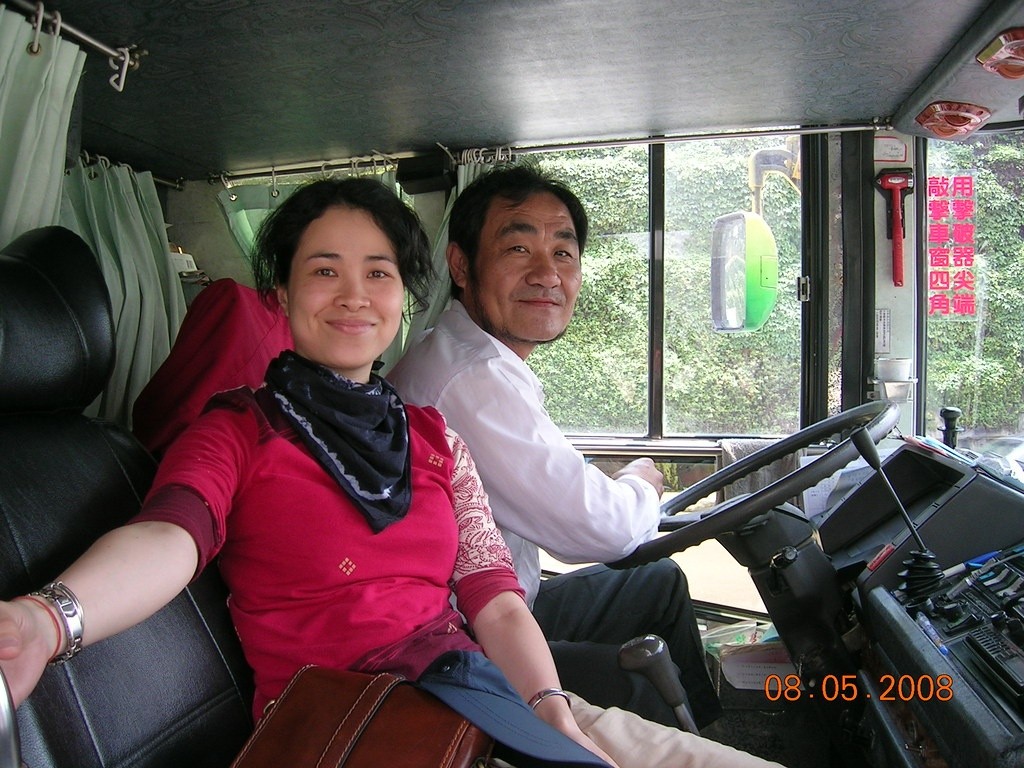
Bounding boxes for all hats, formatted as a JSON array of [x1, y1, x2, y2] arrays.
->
[[418, 649, 613, 768]]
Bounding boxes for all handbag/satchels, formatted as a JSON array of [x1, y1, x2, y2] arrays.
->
[[228, 664, 493, 768]]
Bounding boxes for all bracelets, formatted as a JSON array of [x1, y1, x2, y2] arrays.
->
[[27, 580, 85, 666], [12, 597, 61, 663], [528, 688, 571, 710]]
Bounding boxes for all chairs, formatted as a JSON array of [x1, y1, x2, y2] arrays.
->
[[0, 225, 255, 768], [130, 277, 298, 458]]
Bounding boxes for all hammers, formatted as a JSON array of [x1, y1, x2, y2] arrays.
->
[[876, 174, 914, 288]]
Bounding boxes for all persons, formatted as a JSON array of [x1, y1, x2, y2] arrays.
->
[[382, 164, 782, 757], [0, 176, 790, 768]]
